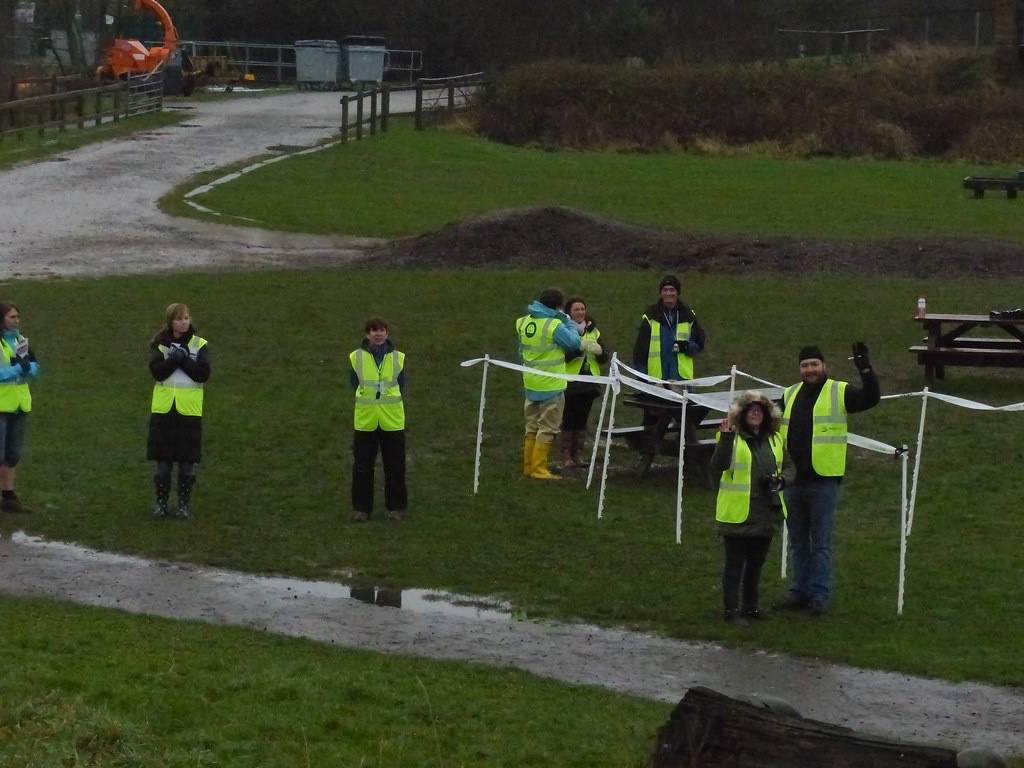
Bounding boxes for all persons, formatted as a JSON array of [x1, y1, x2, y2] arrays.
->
[[633, 275, 707, 432], [0, 303, 41, 514], [516, 288, 581, 481], [778, 341, 881, 613], [710, 388, 797, 627], [147, 303, 211, 519], [348, 317, 407, 524], [560, 297, 609, 466]]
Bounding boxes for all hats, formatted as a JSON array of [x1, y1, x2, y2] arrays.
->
[[659, 275, 682, 296], [799, 346, 824, 364]]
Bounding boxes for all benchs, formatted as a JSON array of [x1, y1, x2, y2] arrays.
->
[[908, 337, 1024, 367], [601, 418, 723, 454]]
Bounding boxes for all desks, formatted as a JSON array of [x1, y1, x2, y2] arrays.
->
[[623, 400, 785, 492], [913, 314, 1024, 386]]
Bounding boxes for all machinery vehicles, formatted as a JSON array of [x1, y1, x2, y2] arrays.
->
[[94, 0, 195, 98]]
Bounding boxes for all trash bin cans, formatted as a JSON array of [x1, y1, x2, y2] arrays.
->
[[295, 39, 340, 92], [340, 35, 388, 91]]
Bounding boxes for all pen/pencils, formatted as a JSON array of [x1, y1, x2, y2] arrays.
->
[[848, 355, 861, 360]]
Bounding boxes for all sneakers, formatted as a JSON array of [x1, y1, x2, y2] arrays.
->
[[1, 493, 34, 515]]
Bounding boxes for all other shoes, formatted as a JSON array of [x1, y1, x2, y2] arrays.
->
[[781, 594, 806, 610], [386, 510, 402, 521], [809, 601, 823, 616], [351, 512, 367, 522], [740, 607, 770, 622], [724, 608, 739, 622]]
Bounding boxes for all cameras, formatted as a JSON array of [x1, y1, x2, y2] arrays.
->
[[760, 475, 783, 511]]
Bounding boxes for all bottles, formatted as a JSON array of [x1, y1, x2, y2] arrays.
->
[[918, 297, 926, 318]]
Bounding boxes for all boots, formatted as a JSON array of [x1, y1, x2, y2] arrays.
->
[[523, 439, 534, 476], [154, 473, 170, 518], [561, 432, 576, 468], [176, 473, 196, 519], [532, 442, 562, 479], [573, 429, 589, 467]]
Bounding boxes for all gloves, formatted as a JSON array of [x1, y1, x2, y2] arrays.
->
[[676, 340, 689, 352], [851, 341, 872, 372], [16, 353, 31, 372], [178, 344, 189, 358], [591, 342, 602, 355], [579, 340, 589, 351]]
